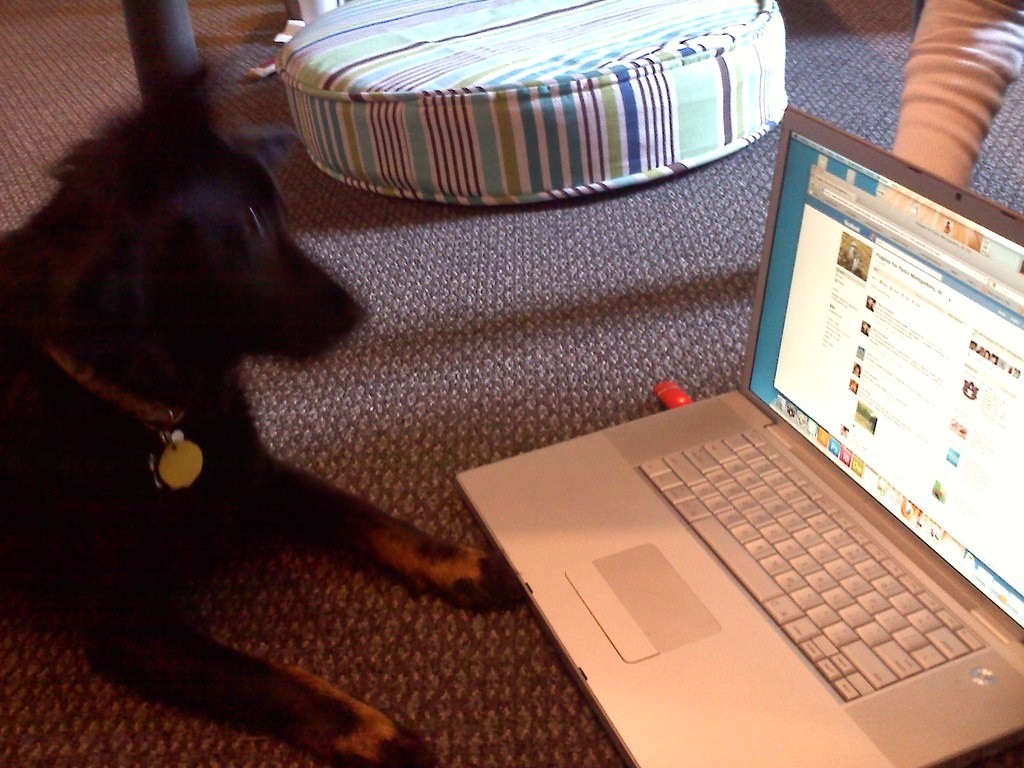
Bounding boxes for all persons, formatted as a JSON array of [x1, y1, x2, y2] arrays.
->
[[893, 0, 1023, 188]]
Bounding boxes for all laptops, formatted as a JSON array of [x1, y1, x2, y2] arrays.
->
[[456, 102, 1023, 768]]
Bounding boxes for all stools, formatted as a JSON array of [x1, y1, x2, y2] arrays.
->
[[275, 0, 788, 203]]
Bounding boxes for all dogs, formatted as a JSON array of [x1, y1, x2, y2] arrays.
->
[[0, 57, 523, 766]]
